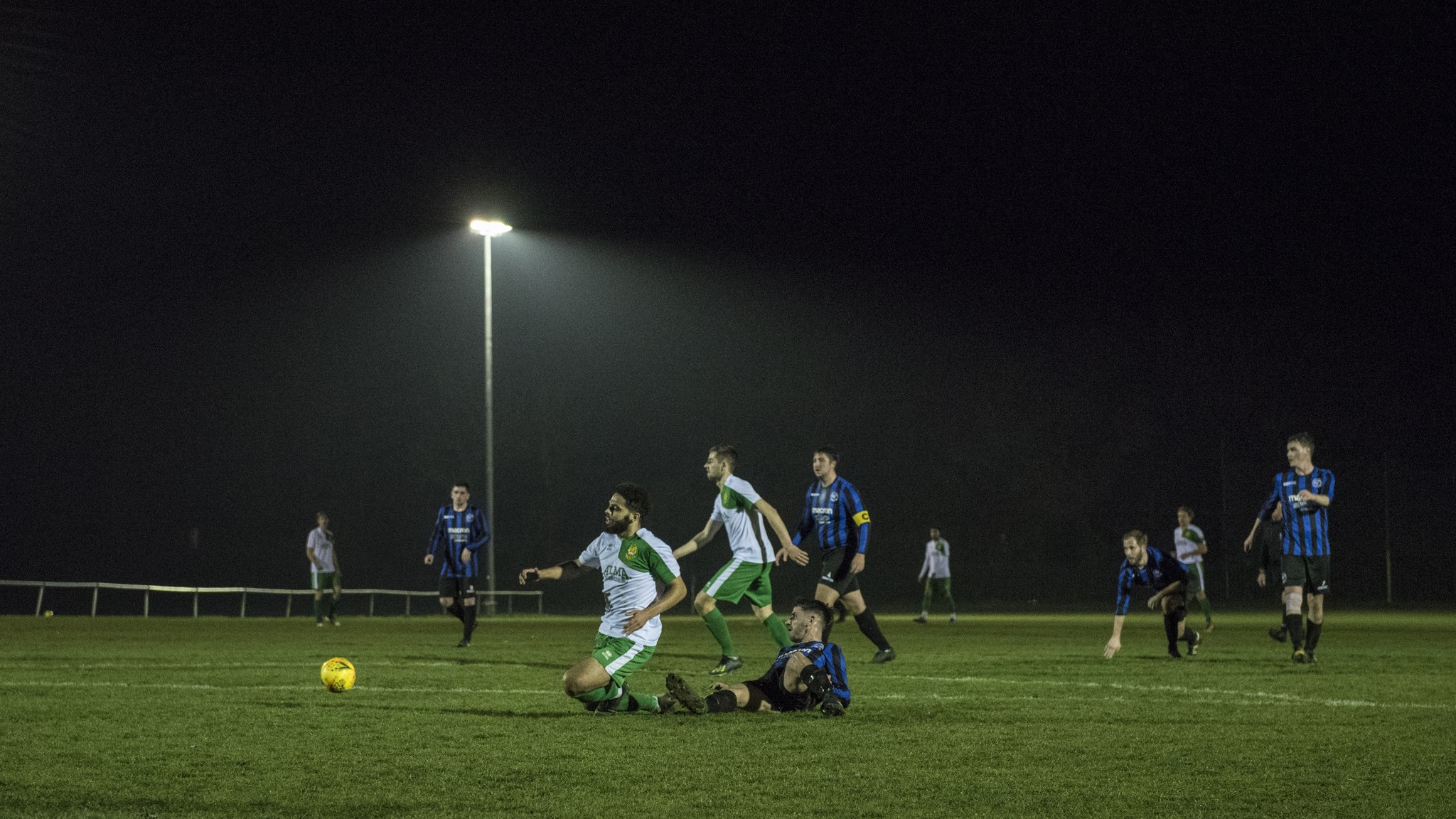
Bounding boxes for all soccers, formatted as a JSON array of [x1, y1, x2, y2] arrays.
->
[[320, 657, 356, 693]]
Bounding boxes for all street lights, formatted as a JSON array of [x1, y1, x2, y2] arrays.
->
[[468, 217, 514, 613]]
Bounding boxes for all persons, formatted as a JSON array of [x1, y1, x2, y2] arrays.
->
[[307, 509, 344, 627], [775, 446, 897, 663], [1103, 529, 1201, 660], [1243, 432, 1336, 663], [665, 597, 851, 717], [424, 480, 490, 648], [674, 444, 809, 677], [518, 484, 688, 713], [1170, 505, 1214, 633], [914, 527, 958, 623], [1257, 501, 1306, 642]]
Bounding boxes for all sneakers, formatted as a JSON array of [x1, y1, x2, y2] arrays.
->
[[1291, 650, 1317, 663], [913, 618, 926, 623], [1204, 623, 1213, 634], [1270, 628, 1287, 643], [950, 618, 956, 623], [325, 616, 339, 626], [872, 646, 896, 664], [820, 699, 845, 718], [458, 638, 470, 647], [1186, 631, 1202, 656], [317, 620, 324, 627], [709, 655, 742, 675], [654, 691, 676, 714], [666, 673, 708, 715]]
